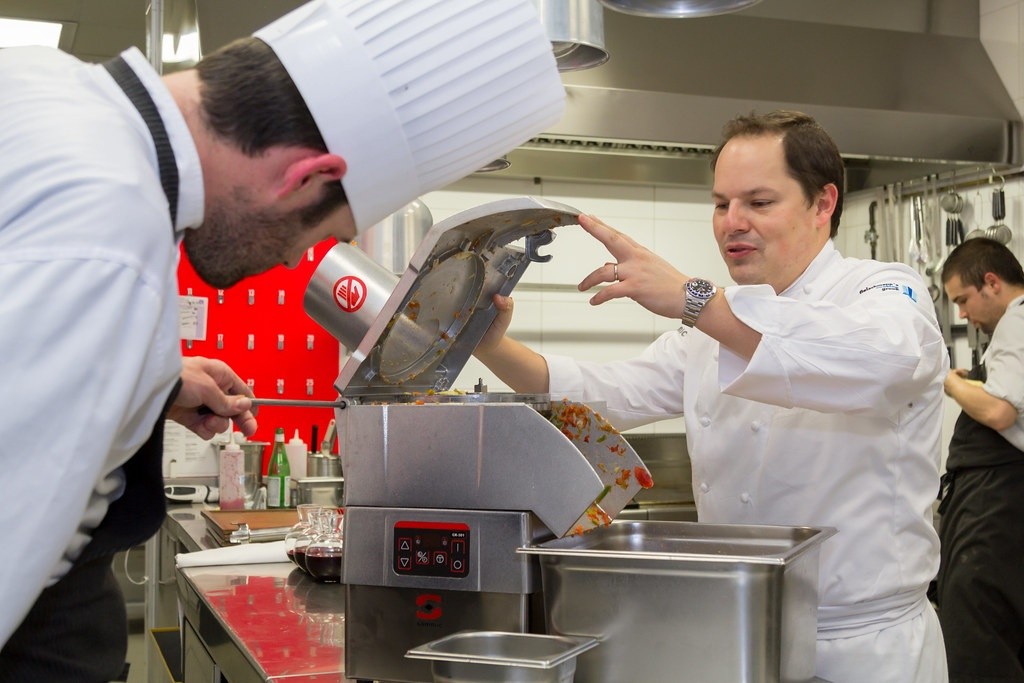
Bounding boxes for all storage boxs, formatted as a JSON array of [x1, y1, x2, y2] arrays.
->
[[148, 626, 184, 683]]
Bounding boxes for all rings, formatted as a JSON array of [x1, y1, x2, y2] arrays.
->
[[614, 264, 620, 280]]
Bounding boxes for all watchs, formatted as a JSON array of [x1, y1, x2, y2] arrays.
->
[[683, 278, 715, 328]]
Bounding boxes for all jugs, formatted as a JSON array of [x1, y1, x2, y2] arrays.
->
[[307, 419, 343, 478]]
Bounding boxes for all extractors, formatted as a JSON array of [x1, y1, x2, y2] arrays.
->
[[0, 0, 1024, 204]]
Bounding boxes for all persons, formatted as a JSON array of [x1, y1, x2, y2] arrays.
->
[[932, 238, 1024, 683], [1, 0, 574, 682], [473, 110, 948, 683]]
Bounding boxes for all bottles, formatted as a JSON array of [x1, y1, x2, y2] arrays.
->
[[285, 428, 307, 491], [266, 427, 291, 509], [219, 432, 245, 510], [284, 504, 343, 584]]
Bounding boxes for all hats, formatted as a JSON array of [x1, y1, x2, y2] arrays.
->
[[251, 0, 564, 237]]
